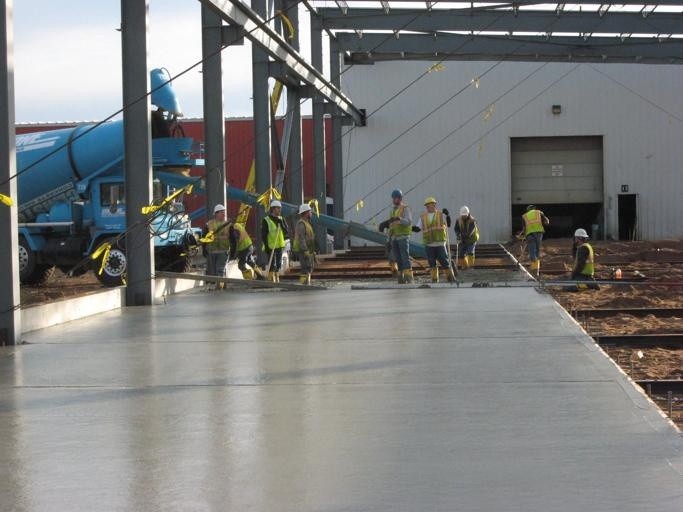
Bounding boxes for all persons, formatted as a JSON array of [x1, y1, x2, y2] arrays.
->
[[453, 206, 478, 270], [290, 203, 316, 285], [520, 204, 549, 277], [228, 223, 263, 281], [411, 197, 456, 283], [201, 204, 231, 283], [258, 200, 288, 282], [566, 228, 600, 291], [385, 188, 412, 284]]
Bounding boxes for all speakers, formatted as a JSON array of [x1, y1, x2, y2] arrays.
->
[[298, 274, 311, 284], [579, 283, 601, 291], [243, 269, 254, 280], [396, 270, 414, 284], [268, 272, 279, 282], [531, 260, 541, 276], [253, 265, 263, 279], [432, 268, 439, 283], [446, 268, 455, 281], [463, 256, 474, 268]]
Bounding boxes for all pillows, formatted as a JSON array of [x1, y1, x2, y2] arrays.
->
[[299, 205, 311, 214], [391, 190, 401, 197], [214, 204, 226, 212], [424, 198, 436, 205], [461, 206, 469, 216], [271, 201, 281, 207], [575, 229, 588, 238]]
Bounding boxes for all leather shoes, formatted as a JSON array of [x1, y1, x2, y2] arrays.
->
[[265, 245, 269, 251], [277, 219, 283, 225], [442, 208, 449, 215], [304, 250, 309, 257]]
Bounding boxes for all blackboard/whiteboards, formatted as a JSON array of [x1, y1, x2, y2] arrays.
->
[[12, 63, 425, 290]]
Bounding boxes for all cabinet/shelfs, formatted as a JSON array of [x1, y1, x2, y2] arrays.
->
[[610, 266, 623, 280]]
